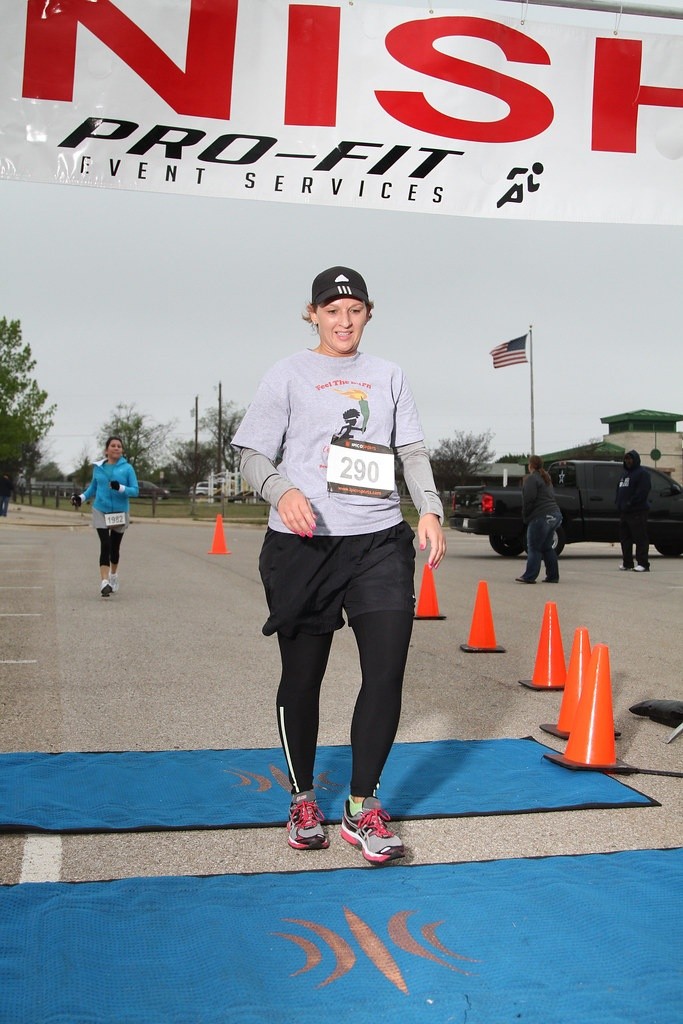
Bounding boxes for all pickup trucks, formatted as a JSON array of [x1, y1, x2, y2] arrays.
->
[[448, 459, 683, 559]]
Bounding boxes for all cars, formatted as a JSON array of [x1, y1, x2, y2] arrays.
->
[[188, 482, 221, 500], [137, 481, 171, 500]]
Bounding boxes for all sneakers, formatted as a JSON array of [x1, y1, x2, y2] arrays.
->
[[101, 579, 112, 597], [341, 796, 405, 862], [110, 572, 118, 592], [286, 791, 329, 849]]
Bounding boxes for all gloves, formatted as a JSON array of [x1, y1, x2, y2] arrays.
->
[[70, 493, 81, 506], [109, 481, 119, 490]]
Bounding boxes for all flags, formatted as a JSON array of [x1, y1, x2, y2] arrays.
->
[[489, 333, 529, 368]]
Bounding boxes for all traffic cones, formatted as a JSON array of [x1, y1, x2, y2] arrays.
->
[[539, 626, 622, 741], [541, 644, 639, 776], [460, 580, 506, 652], [412, 563, 448, 619], [518, 602, 568, 692], [207, 513, 230, 553]]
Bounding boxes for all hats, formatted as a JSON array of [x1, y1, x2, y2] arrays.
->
[[312, 266, 368, 305]]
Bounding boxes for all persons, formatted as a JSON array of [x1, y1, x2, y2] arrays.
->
[[515, 455, 563, 584], [231, 265, 444, 865], [0, 474, 12, 516], [615, 449, 651, 572], [71, 437, 140, 597]]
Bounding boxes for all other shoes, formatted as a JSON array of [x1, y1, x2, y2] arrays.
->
[[633, 565, 646, 571], [542, 578, 559, 583], [620, 565, 629, 570], [515, 577, 537, 584]]
